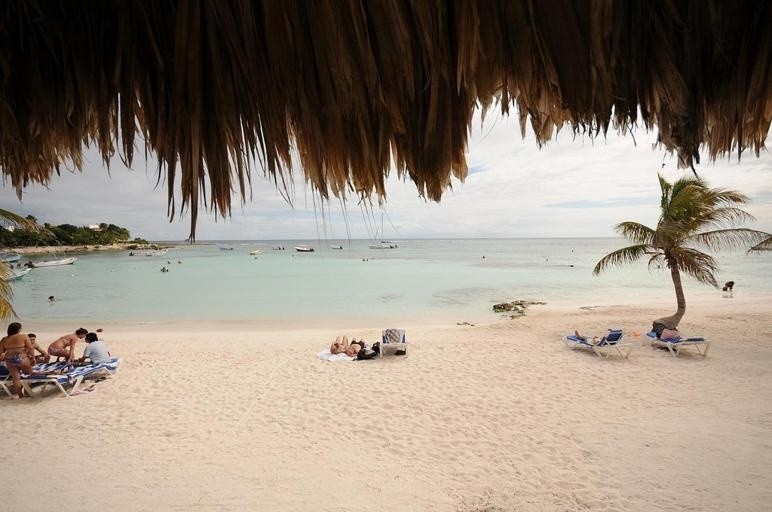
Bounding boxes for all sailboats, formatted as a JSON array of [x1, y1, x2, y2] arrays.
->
[[368, 212, 399, 249]]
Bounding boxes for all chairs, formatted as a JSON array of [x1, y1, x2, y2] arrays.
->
[[646, 331, 713, 358], [0, 356, 121, 399], [380, 328, 408, 359], [567, 330, 634, 360]]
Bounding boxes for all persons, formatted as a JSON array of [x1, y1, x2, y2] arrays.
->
[[47, 328, 88, 370], [25, 333, 50, 365], [723, 281, 734, 291], [345, 337, 365, 357], [47, 295, 56, 302], [656, 325, 680, 342], [0, 323, 37, 398], [77, 332, 111, 364], [9, 260, 35, 269], [574, 328, 614, 346], [331, 335, 347, 354]]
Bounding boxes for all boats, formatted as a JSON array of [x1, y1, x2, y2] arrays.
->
[[25, 256, 77, 269], [272, 244, 286, 250], [219, 247, 234, 250], [129, 248, 168, 258], [295, 246, 314, 252], [1, 264, 31, 281], [330, 244, 344, 250], [250, 250, 265, 255], [0, 249, 24, 263]]
